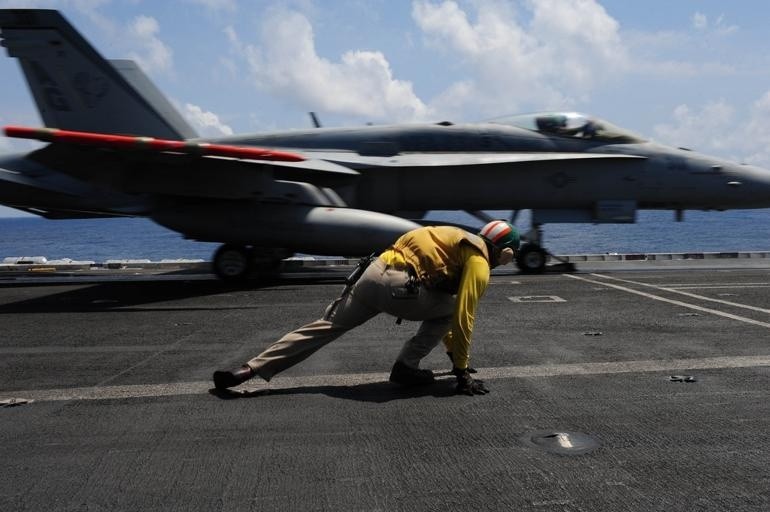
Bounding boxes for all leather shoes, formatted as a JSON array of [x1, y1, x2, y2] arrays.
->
[[213, 365, 254, 389], [389, 369, 434, 382]]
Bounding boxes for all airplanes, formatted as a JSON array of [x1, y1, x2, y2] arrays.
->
[[0, 8, 770, 283]]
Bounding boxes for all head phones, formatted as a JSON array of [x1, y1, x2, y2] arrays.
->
[[478, 232, 513, 266]]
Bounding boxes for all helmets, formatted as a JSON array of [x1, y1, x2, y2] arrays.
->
[[478, 219, 520, 251]]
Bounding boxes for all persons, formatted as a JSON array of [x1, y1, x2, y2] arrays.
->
[[213, 218, 520, 397], [537, 115, 598, 135]]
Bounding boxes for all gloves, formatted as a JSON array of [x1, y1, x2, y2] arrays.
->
[[451, 366, 490, 396]]
